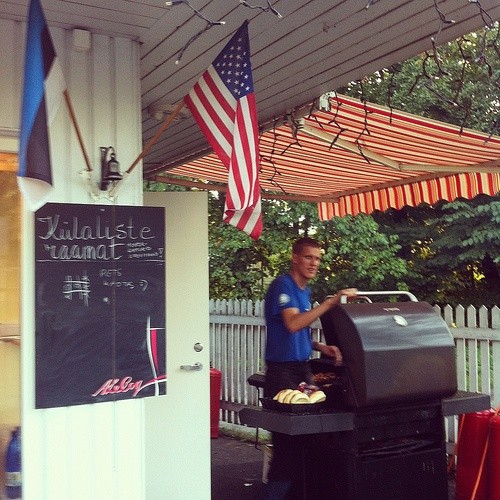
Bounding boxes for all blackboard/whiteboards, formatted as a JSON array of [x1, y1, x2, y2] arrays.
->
[[33, 201, 167, 409]]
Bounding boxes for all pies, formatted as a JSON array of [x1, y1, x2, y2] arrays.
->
[[273, 389, 326, 404]]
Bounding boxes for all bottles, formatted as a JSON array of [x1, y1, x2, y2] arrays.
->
[[262, 443, 273, 484]]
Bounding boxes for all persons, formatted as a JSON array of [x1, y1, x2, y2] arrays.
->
[[260, 237, 359, 500]]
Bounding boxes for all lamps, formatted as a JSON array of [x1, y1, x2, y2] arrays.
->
[[100, 146, 123, 190]]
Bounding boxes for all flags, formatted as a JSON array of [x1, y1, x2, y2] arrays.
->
[[16, 0, 67, 213], [184, 22, 265, 241]]
